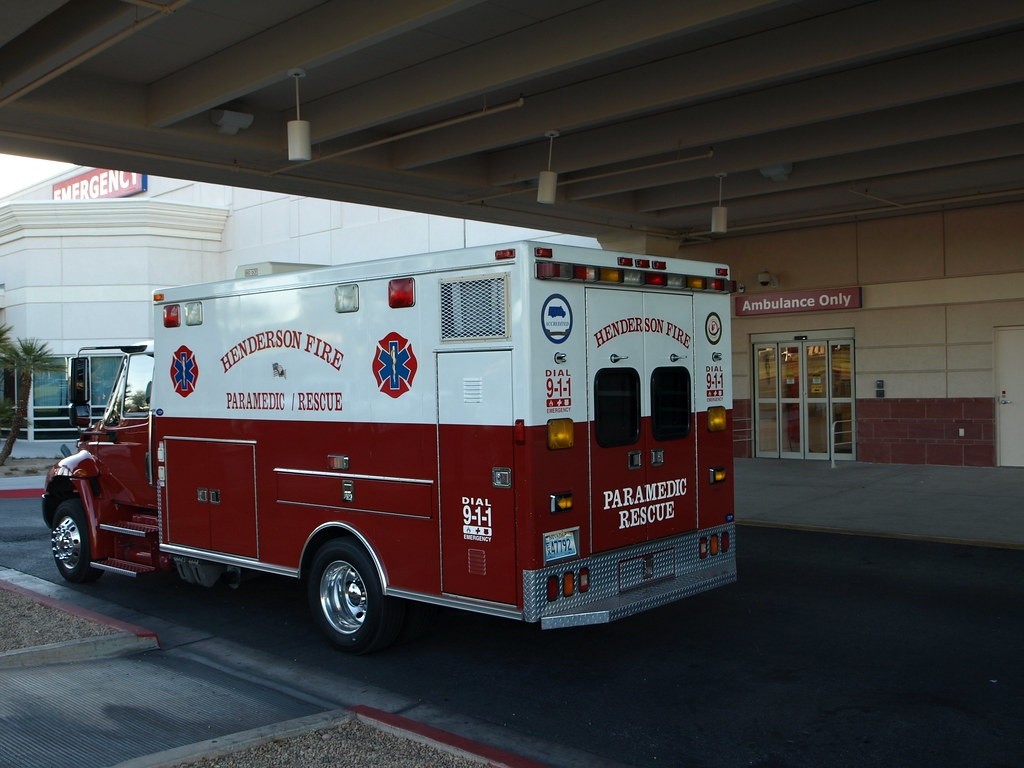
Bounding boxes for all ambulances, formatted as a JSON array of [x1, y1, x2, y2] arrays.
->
[[40, 238, 740, 658]]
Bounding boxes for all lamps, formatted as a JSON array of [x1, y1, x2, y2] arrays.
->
[[288, 68, 312, 162], [536, 130, 560, 205], [711, 172, 727, 234]]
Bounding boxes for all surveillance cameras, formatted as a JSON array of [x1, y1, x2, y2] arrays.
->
[[757, 271, 771, 285]]
